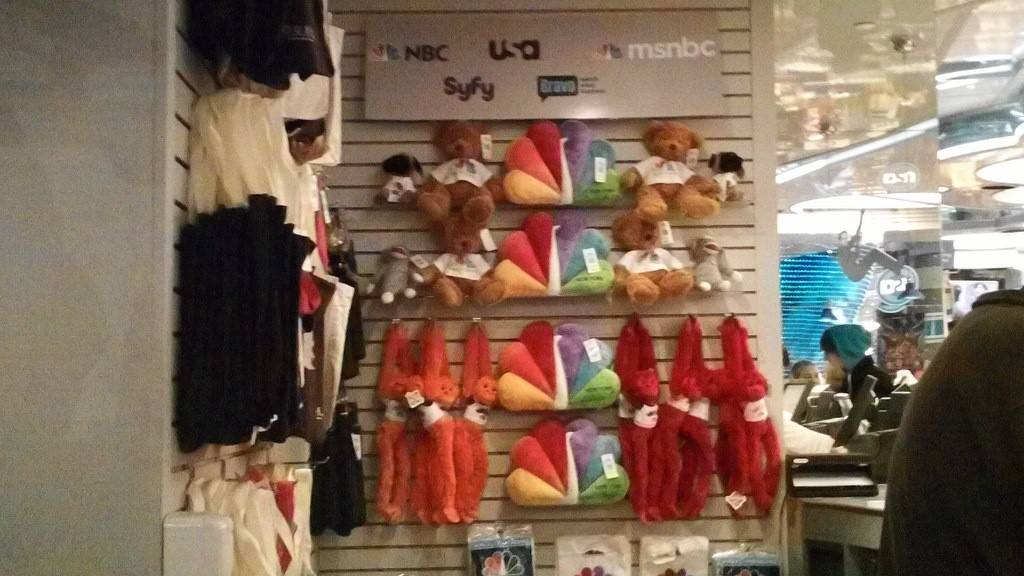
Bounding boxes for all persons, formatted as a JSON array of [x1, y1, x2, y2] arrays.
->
[[952, 282, 988, 320], [879, 284, 1024, 576], [789, 323, 892, 420]]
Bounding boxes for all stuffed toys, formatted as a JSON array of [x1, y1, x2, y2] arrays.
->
[[367, 122, 783, 527]]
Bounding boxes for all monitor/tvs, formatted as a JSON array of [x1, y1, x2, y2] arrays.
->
[[833, 375, 878, 450], [783, 379, 814, 423]]
